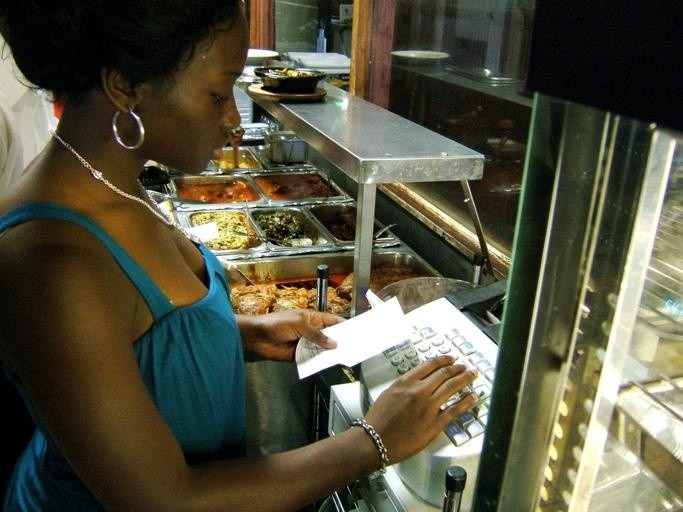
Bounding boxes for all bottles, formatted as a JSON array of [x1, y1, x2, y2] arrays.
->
[[316, 27, 327, 53]]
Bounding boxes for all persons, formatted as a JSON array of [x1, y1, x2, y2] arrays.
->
[[0, 0, 477, 511]]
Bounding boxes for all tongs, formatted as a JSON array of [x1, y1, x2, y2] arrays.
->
[[375, 222, 399, 242]]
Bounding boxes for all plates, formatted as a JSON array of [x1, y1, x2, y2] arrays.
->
[[390, 48, 452, 66]]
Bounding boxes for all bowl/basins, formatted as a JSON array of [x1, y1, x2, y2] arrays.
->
[[252, 64, 328, 94], [244, 48, 280, 64], [370, 276, 481, 315]]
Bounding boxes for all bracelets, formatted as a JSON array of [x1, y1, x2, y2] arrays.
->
[[352, 419, 389, 472]]
